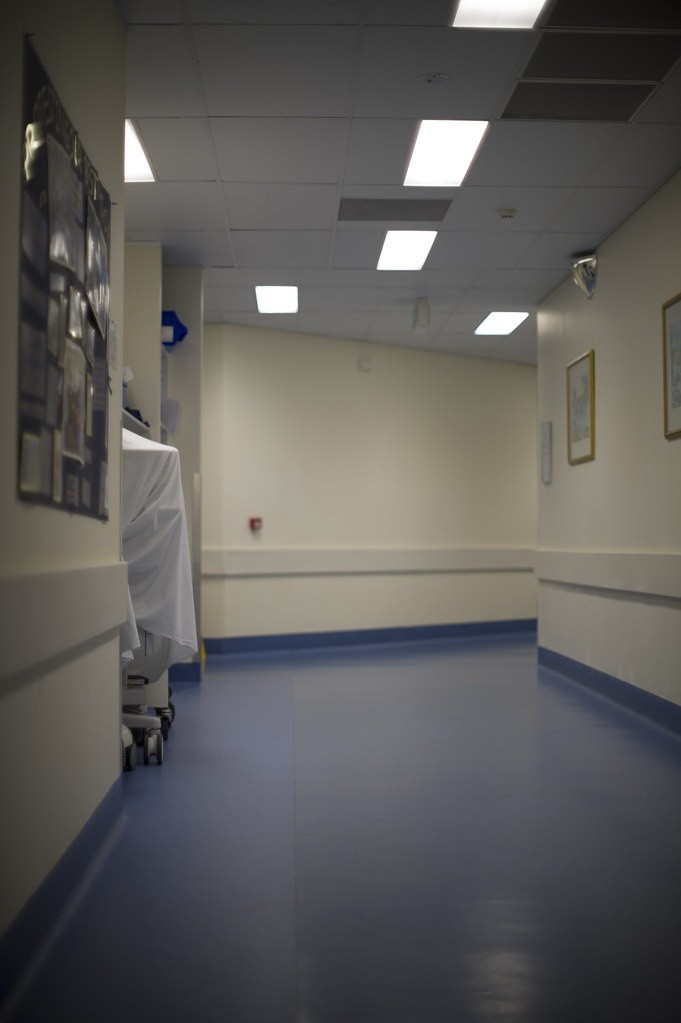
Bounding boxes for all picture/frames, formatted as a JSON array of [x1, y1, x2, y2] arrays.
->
[[565, 349, 595, 465], [661, 293, 681, 440]]
